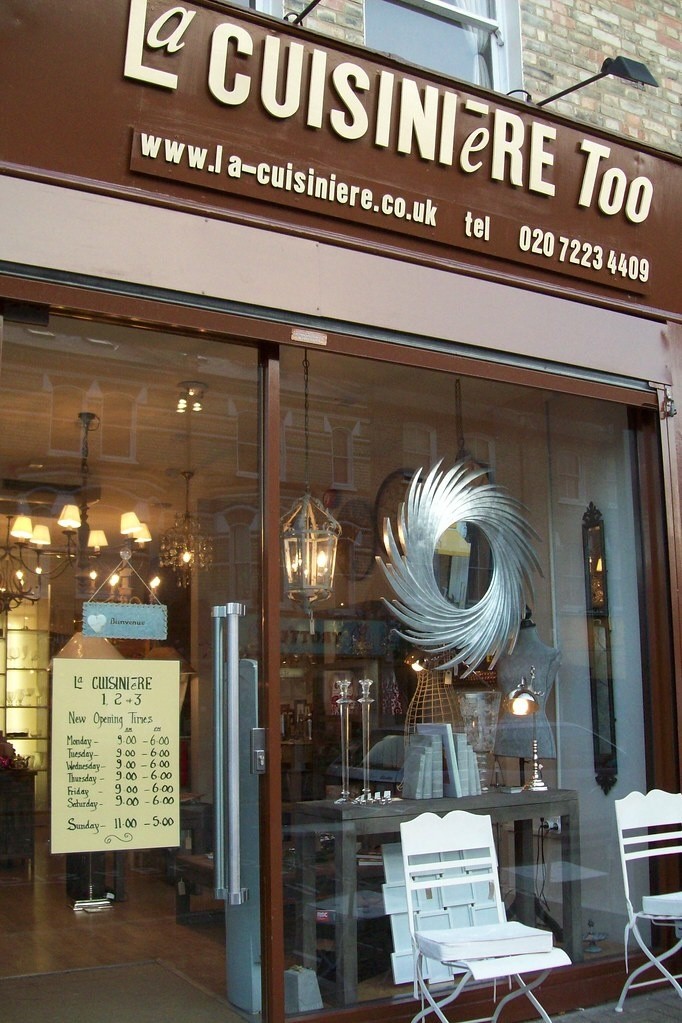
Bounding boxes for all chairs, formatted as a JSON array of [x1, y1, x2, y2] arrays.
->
[[615, 789, 682, 1012], [399, 809, 573, 1023]]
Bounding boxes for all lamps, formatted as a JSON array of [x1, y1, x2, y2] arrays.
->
[[503, 686, 549, 793], [0, 410, 163, 642], [161, 380, 213, 588], [538, 56, 658, 107], [277, 349, 341, 604]]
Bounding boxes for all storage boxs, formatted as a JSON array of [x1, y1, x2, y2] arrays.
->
[[543, 815, 562, 834]]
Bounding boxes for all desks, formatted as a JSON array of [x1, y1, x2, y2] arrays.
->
[[280, 788, 581, 1009], [170, 853, 223, 924], [0, 959, 258, 1023], [0, 769, 38, 869], [179, 803, 212, 850]]
